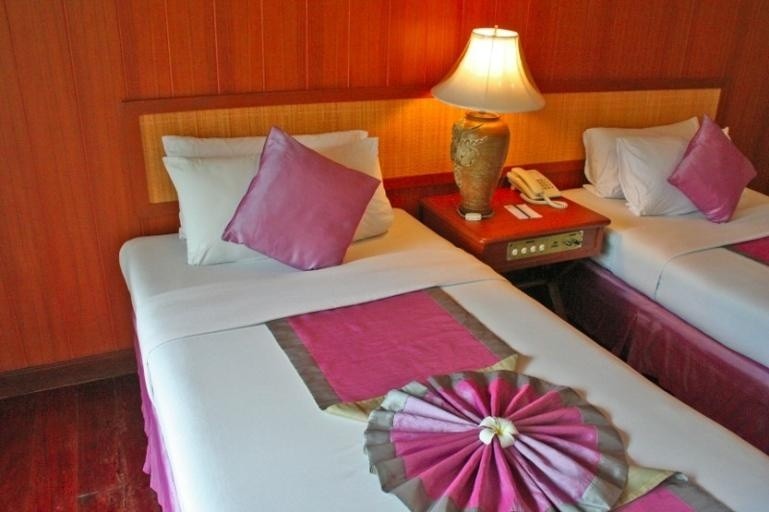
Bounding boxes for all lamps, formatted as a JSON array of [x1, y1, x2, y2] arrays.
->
[[426, 22, 548, 217]]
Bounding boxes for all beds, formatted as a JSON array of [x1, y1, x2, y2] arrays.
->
[[112, 180, 767, 512]]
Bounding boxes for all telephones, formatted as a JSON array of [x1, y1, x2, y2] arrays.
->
[[507, 167, 561, 199]]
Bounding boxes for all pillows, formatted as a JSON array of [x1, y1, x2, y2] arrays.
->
[[159, 128, 398, 266], [221, 124, 386, 268], [576, 110, 757, 223]]
